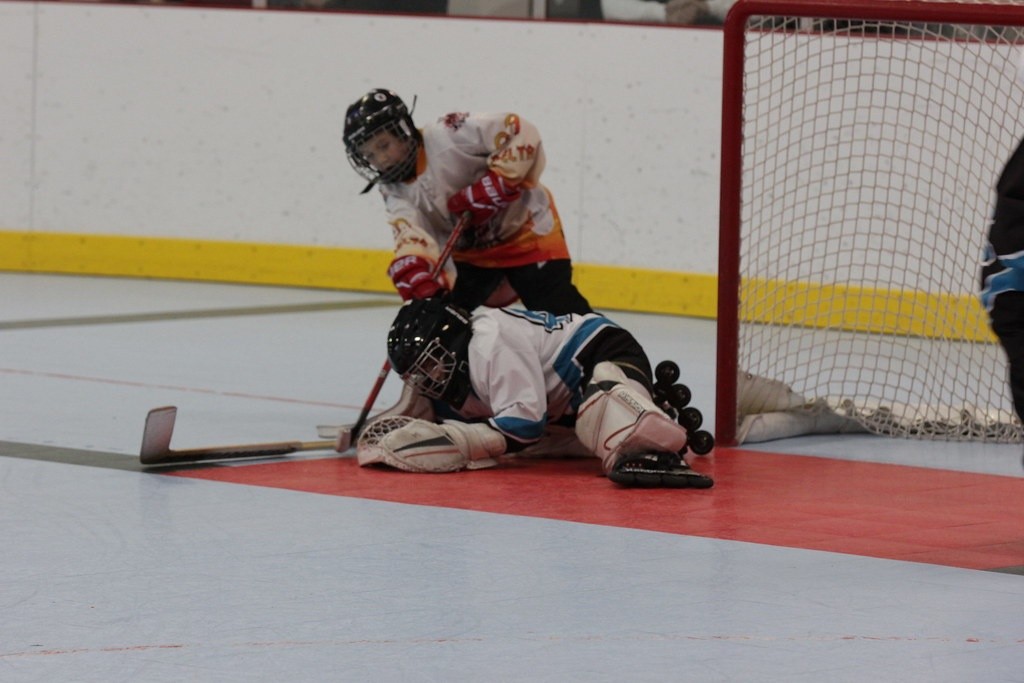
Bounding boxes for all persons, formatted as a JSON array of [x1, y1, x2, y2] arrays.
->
[[342, 89, 716, 488]]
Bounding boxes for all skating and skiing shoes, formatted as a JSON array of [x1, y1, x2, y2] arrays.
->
[[610, 448, 715, 490], [646, 361, 715, 456]]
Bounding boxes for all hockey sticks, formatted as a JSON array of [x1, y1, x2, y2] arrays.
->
[[335, 211, 468, 454], [137, 406, 361, 464]]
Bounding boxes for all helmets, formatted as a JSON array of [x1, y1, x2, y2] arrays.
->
[[343, 89, 425, 184], [387, 297, 474, 412]]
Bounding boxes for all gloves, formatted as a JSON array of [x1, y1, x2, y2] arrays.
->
[[387, 254, 445, 303], [448, 171, 521, 234]]
